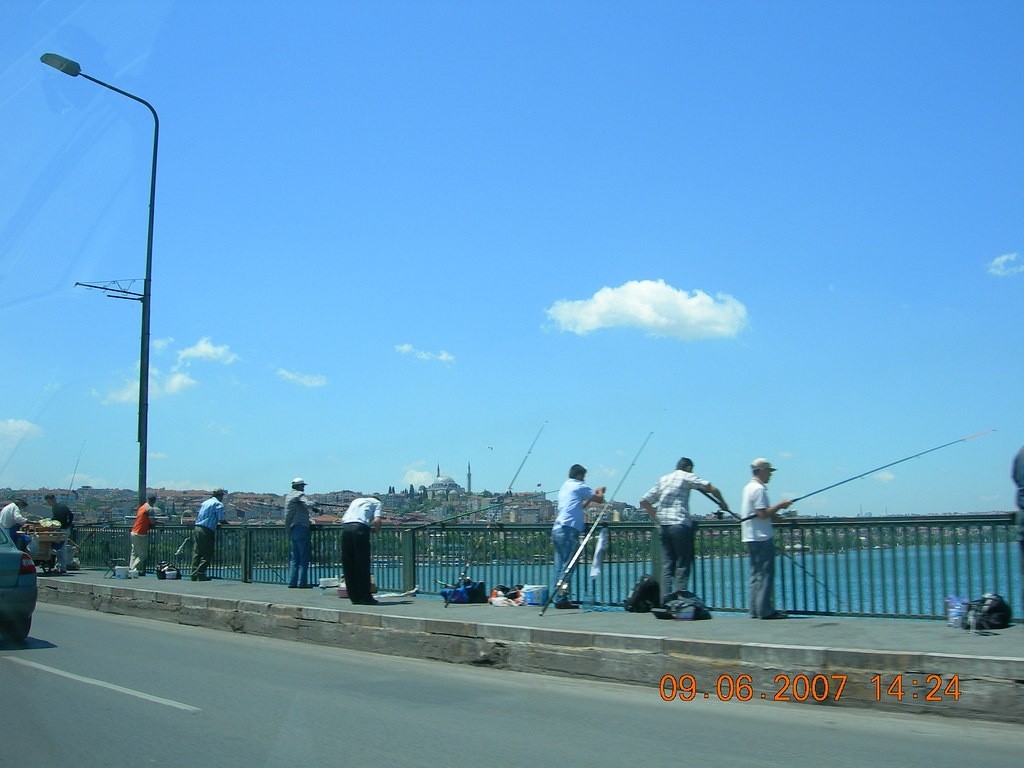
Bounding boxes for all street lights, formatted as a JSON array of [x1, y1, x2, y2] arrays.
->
[[40, 53, 161, 526]]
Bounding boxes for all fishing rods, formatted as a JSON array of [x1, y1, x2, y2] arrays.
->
[[694, 488, 847, 605], [170, 528, 241, 576], [83, 519, 129, 526], [444, 421, 548, 609], [404, 489, 561, 531], [311, 501, 435, 520], [65, 439, 86, 505], [232, 530, 288, 584], [736, 429, 997, 522], [0, 381, 69, 475], [539, 431, 654, 617]]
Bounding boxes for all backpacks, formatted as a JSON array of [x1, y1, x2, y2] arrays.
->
[[625, 575, 659, 612]]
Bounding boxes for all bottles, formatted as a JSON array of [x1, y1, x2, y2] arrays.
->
[[459, 572, 471, 587]]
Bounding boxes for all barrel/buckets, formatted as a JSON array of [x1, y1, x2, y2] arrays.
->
[[523, 585, 548, 607], [166, 571, 177, 579], [114, 565, 130, 579]]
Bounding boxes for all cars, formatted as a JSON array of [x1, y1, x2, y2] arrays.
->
[[0, 524, 38, 643]]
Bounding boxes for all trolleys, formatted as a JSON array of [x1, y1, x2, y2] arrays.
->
[[15, 532, 67, 574]]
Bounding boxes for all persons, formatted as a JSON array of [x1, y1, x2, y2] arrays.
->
[[1013, 445, 1024, 585], [741, 458, 792, 620], [129, 495, 165, 575], [0, 498, 39, 549], [44, 493, 73, 575], [639, 457, 728, 605], [341, 496, 383, 606], [190, 487, 231, 582], [284, 477, 319, 589], [551, 464, 606, 609]]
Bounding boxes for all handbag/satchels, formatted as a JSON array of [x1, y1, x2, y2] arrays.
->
[[441, 580, 486, 604], [963, 591, 1010, 631]]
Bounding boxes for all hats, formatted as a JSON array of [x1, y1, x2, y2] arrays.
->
[[15, 496, 29, 507], [750, 458, 777, 473], [293, 478, 308, 487], [213, 487, 226, 496]]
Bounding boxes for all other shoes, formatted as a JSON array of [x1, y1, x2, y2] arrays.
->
[[289, 582, 314, 588], [191, 574, 211, 581], [762, 609, 786, 620], [352, 596, 379, 606], [555, 601, 579, 610], [49, 569, 66, 574]]
